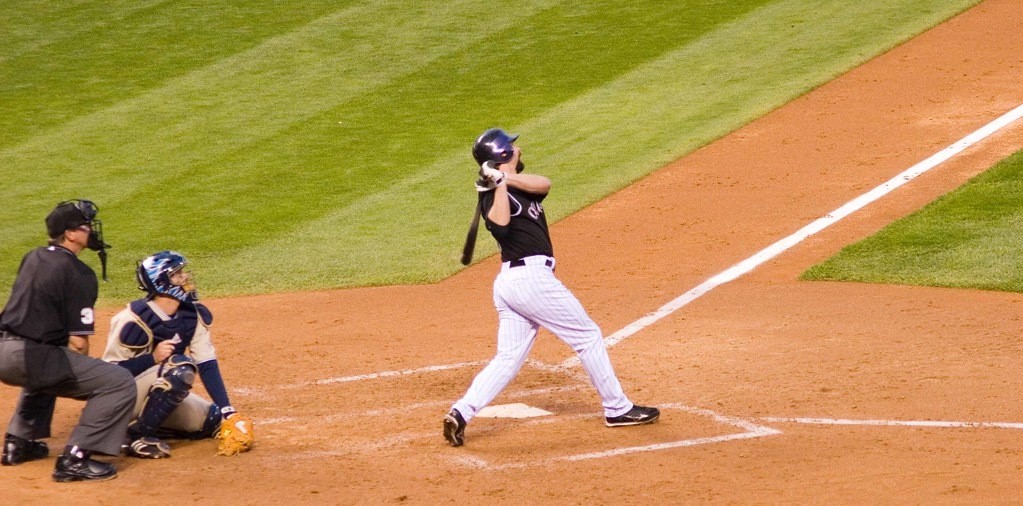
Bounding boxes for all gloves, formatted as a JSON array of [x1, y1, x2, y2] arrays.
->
[[481, 160, 506, 186], [475, 170, 499, 192]]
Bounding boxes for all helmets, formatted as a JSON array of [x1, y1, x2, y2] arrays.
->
[[45, 197, 90, 239], [472, 127, 521, 164], [136, 250, 201, 303]]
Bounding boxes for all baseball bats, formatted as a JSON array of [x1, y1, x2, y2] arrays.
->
[[459, 159, 499, 268]]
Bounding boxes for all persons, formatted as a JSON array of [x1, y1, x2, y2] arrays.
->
[[103, 251, 252, 461], [0, 198, 136, 482], [442, 128, 659, 446]]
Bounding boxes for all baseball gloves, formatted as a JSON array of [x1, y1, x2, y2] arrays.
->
[[214, 413, 256, 458]]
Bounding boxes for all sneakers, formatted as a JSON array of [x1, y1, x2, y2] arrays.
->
[[442, 410, 467, 446], [1, 432, 50, 465], [122, 436, 170, 459], [605, 404, 662, 426], [52, 455, 118, 483]]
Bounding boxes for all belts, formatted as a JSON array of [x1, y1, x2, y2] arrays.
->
[[509, 260, 552, 268]]
